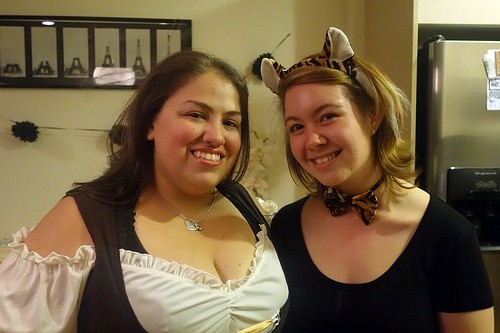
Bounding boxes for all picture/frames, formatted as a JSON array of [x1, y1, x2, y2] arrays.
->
[[0, 15, 192, 90]]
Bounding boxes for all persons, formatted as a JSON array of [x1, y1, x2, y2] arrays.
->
[[260, 26, 494, 333], [0, 51, 293, 332]]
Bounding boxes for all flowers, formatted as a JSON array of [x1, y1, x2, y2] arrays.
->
[[236, 132, 278, 199]]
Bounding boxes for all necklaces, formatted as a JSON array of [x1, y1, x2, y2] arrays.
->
[[153, 187, 216, 231]]
